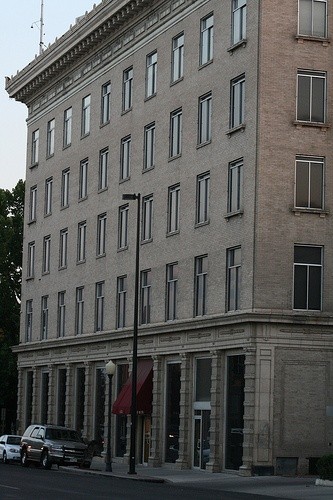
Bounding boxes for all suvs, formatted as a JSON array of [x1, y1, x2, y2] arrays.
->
[[19, 423, 91, 470]]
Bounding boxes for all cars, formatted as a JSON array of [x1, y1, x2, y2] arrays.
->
[[0, 434, 23, 464]]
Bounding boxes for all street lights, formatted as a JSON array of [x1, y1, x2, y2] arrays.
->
[[105, 360, 117, 473]]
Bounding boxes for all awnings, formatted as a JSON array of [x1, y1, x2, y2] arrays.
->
[[112, 360, 152, 415]]
